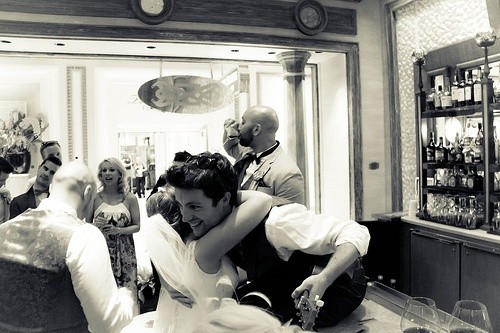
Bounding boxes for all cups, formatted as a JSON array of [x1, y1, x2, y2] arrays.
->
[[421, 195, 500, 233], [448, 300, 493, 333], [399, 296, 442, 333]]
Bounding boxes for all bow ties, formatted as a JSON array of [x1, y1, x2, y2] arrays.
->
[[35, 187, 49, 196], [245, 140, 281, 166]]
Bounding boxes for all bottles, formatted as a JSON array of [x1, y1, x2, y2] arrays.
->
[[425, 66, 500, 192]]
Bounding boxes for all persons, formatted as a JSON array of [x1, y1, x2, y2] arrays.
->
[[0, 106, 371, 333]]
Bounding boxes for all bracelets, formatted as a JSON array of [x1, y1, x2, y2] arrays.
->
[[118, 227, 121, 236]]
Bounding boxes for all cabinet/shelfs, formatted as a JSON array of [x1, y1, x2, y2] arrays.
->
[[399, 34, 500, 333]]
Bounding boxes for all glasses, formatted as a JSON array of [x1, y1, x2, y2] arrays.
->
[[173, 151, 219, 169]]
